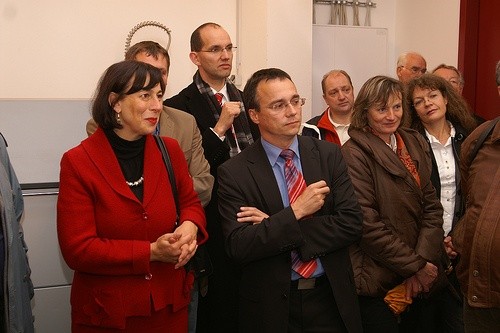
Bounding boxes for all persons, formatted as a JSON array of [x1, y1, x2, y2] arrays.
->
[[299, 68, 356, 150], [162, 22, 266, 333], [86, 42, 214, 207], [215, 67, 364, 333], [340, 74, 445, 332], [431, 65, 465, 99], [451, 60, 500, 333], [56, 61, 207, 333], [0, 133, 35, 333], [397, 52, 426, 86], [401, 75, 470, 279]]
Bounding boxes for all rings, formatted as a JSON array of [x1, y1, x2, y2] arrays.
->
[[418, 285, 423, 289]]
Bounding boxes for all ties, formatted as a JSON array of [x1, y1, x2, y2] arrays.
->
[[215, 92, 224, 108], [279, 149, 318, 279]]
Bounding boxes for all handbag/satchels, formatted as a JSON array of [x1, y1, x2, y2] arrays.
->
[[190, 247, 211, 281]]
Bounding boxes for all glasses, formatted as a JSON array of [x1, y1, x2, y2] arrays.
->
[[199, 47, 237, 55], [258, 98, 307, 111], [401, 65, 426, 75]]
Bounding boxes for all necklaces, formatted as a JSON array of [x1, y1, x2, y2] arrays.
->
[[432, 136, 442, 144], [124, 173, 147, 186]]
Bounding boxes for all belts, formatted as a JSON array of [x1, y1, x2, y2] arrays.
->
[[290, 276, 328, 290]]
